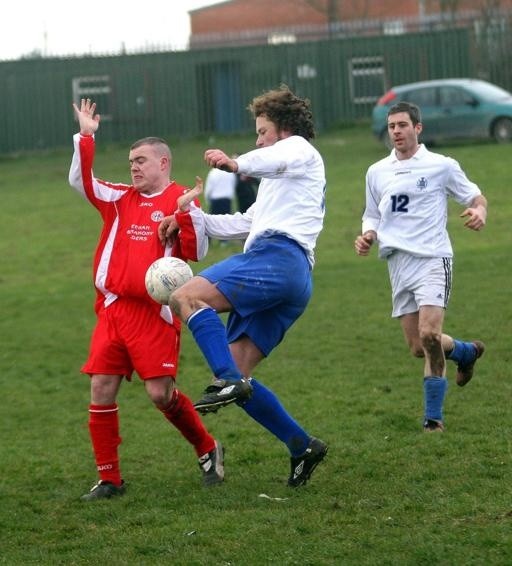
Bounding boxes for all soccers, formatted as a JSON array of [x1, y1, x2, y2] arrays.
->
[[145, 256, 193, 305]]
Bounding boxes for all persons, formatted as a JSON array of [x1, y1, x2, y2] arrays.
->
[[202, 162, 235, 249], [165, 88, 332, 490], [64, 97, 229, 502], [350, 103, 490, 430], [232, 152, 260, 210]]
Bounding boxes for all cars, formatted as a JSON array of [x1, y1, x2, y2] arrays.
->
[[369, 75, 512, 153]]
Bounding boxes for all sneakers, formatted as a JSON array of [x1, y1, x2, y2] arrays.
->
[[457, 342, 484, 387], [198, 440, 225, 486], [79, 479, 127, 501], [194, 378, 252, 415], [288, 439, 329, 487], [422, 418, 446, 432]]
